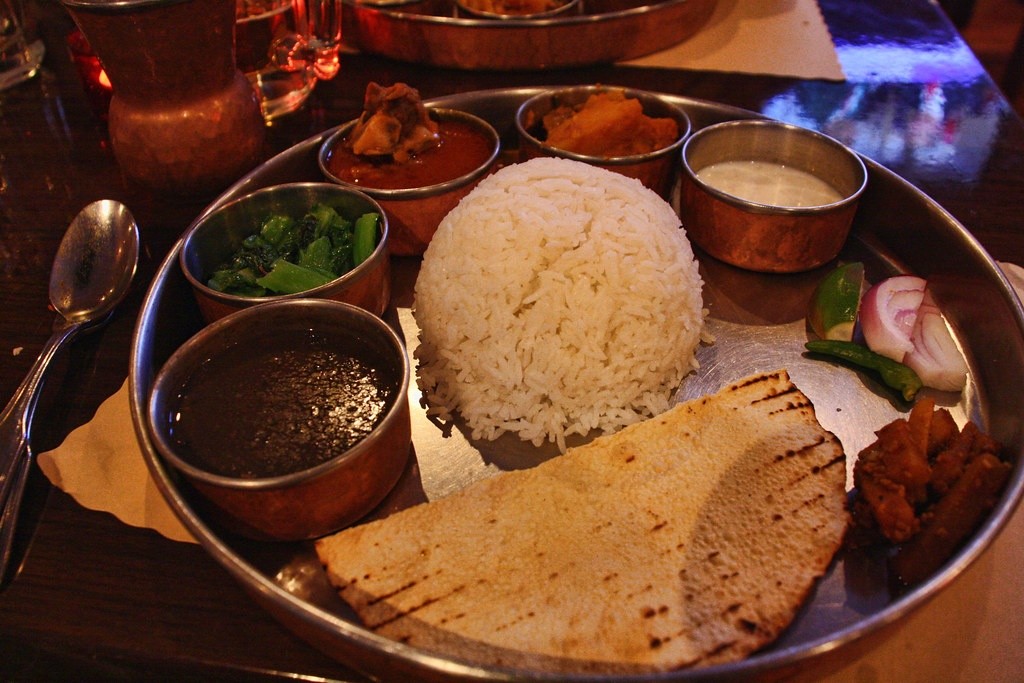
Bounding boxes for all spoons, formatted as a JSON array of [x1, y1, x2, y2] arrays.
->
[[1, 201, 139, 579]]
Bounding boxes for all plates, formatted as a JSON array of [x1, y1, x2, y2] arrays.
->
[[350, 1, 715, 68], [127, 84, 1022, 682]]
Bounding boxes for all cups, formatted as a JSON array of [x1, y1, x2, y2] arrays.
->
[[0, 1, 342, 125]]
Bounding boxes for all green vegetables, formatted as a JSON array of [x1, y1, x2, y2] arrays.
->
[[208, 206, 379, 296]]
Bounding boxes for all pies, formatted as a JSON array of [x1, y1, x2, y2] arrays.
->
[[315, 371, 848, 677]]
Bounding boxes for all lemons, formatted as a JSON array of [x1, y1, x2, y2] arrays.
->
[[807, 261, 865, 341]]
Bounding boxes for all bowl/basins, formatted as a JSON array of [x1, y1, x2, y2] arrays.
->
[[454, 1, 579, 21], [180, 183, 390, 325], [147, 299, 410, 543], [515, 86, 691, 191], [681, 120, 868, 272], [319, 106, 500, 257]]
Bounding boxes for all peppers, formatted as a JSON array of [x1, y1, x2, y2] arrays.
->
[[804, 341, 922, 400]]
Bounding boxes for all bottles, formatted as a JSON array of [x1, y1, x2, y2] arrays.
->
[[62, 0, 268, 190]]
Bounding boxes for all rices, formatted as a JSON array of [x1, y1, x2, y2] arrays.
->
[[412, 157, 717, 454]]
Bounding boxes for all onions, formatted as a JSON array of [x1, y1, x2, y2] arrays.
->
[[860, 276, 970, 392]]
